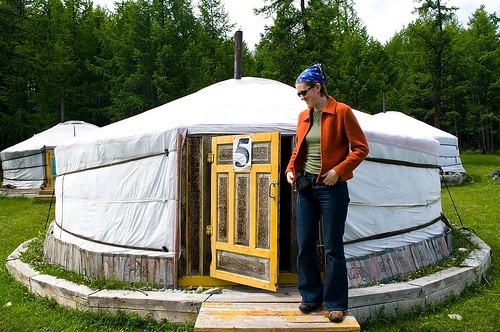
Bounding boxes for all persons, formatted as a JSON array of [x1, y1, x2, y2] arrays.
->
[[285, 64, 369, 322]]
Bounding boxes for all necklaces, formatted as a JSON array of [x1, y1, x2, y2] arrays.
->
[[315, 106, 325, 123]]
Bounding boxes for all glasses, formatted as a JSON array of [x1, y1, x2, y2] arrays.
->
[[297, 81, 316, 97]]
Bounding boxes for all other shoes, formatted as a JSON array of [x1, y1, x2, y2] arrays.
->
[[298, 304, 321, 311], [328, 311, 343, 322]]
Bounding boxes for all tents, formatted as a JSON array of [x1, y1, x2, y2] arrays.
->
[[0, 121, 102, 189], [45, 77, 451, 287], [373, 111, 466, 175]]
